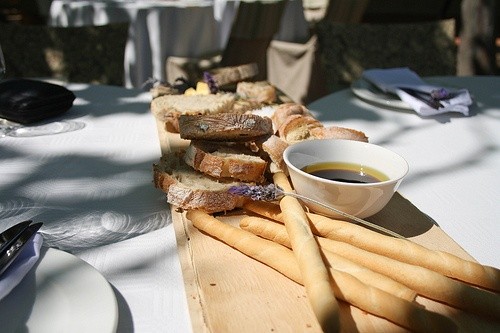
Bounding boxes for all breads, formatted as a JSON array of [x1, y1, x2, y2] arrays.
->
[[205, 61, 258, 88], [150, 94, 369, 213], [237, 80, 277, 104]]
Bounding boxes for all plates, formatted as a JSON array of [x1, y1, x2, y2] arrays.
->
[[0, 246, 119, 333], [351, 79, 414, 113]]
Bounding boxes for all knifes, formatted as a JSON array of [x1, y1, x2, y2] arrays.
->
[[0, 220, 43, 276]]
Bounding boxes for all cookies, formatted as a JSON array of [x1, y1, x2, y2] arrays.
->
[[183, 173, 500, 333]]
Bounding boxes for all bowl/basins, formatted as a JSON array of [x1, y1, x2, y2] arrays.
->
[[282, 139, 409, 224]]
